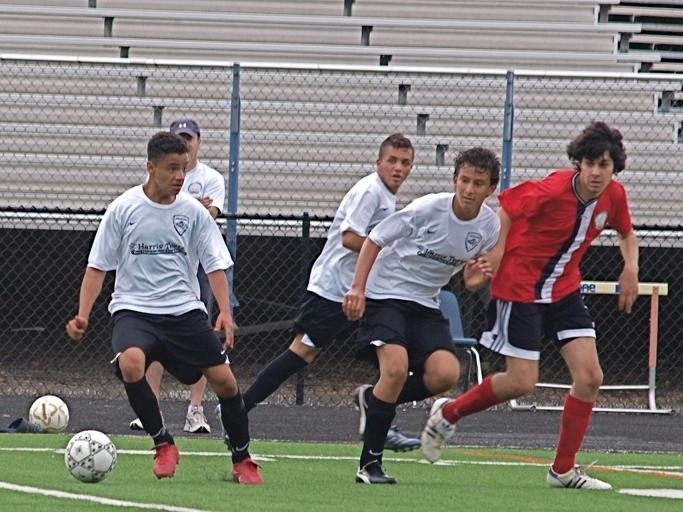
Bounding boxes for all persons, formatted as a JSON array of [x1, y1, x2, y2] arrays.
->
[[341, 147, 501, 485], [420, 121, 640, 491], [128, 120, 225, 437], [215, 133, 423, 454], [66, 132, 264, 484]]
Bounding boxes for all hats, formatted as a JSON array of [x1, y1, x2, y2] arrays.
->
[[170, 119, 201, 139]]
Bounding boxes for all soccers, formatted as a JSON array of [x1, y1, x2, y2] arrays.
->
[[28, 395, 69, 434], [64, 430, 114, 483]]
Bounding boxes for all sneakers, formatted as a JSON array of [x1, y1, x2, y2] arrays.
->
[[153, 442, 179, 478], [354, 462, 395, 485], [383, 426, 419, 451], [215, 404, 228, 440], [232, 459, 264, 486], [130, 413, 167, 431], [354, 385, 371, 439], [186, 405, 212, 433], [547, 465, 613, 492], [423, 399, 458, 463]]
